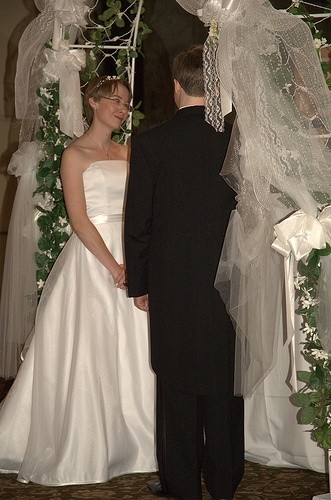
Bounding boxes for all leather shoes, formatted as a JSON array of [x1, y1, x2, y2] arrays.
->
[[148, 481, 170, 497]]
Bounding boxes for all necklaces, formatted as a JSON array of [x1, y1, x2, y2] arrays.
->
[[84, 131, 112, 158]]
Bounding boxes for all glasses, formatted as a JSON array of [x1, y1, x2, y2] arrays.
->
[[100, 96, 132, 112]]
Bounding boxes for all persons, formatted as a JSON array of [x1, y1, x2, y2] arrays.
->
[[0, 76, 160, 488], [123, 45, 247, 500]]
